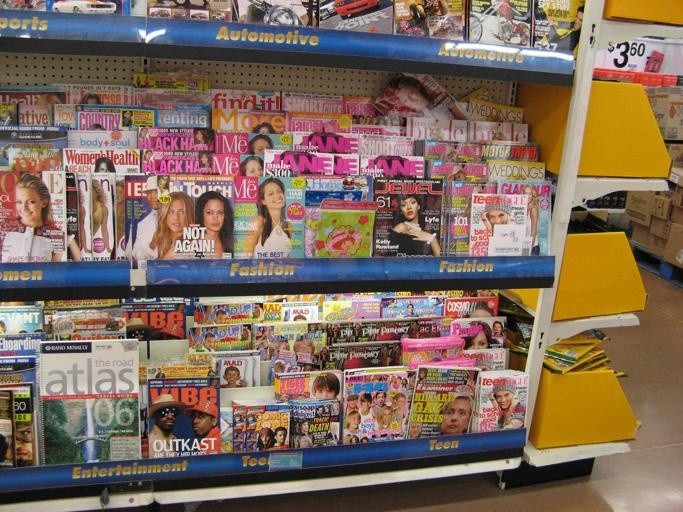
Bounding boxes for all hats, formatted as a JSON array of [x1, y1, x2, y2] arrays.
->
[[184, 400, 219, 418], [493, 377, 516, 395], [485, 196, 511, 215], [149, 394, 186, 419]]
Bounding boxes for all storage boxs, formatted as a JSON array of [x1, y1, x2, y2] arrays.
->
[[607, 35, 683, 265]]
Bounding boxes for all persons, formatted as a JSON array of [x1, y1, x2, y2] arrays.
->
[[1, 173, 65, 264], [272, 428, 291, 451], [546, 5, 585, 52], [393, 77, 468, 123], [244, 178, 294, 261], [255, 425, 274, 450], [150, 190, 197, 261], [521, 185, 542, 256], [186, 398, 222, 458], [459, 321, 492, 352], [311, 372, 341, 405], [436, 391, 475, 435], [147, 393, 187, 461], [192, 191, 233, 260], [386, 195, 441, 257], [488, 376, 526, 433], [478, 194, 519, 238]]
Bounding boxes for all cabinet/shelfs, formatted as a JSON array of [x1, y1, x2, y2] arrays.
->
[[0, 1, 670, 510]]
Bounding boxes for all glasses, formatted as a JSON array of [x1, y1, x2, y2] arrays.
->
[[154, 407, 177, 417]]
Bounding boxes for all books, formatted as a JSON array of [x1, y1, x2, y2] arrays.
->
[[0, 74, 550, 266], [0, 285, 531, 471], [1, 0, 585, 53], [542, 326, 628, 379]]
